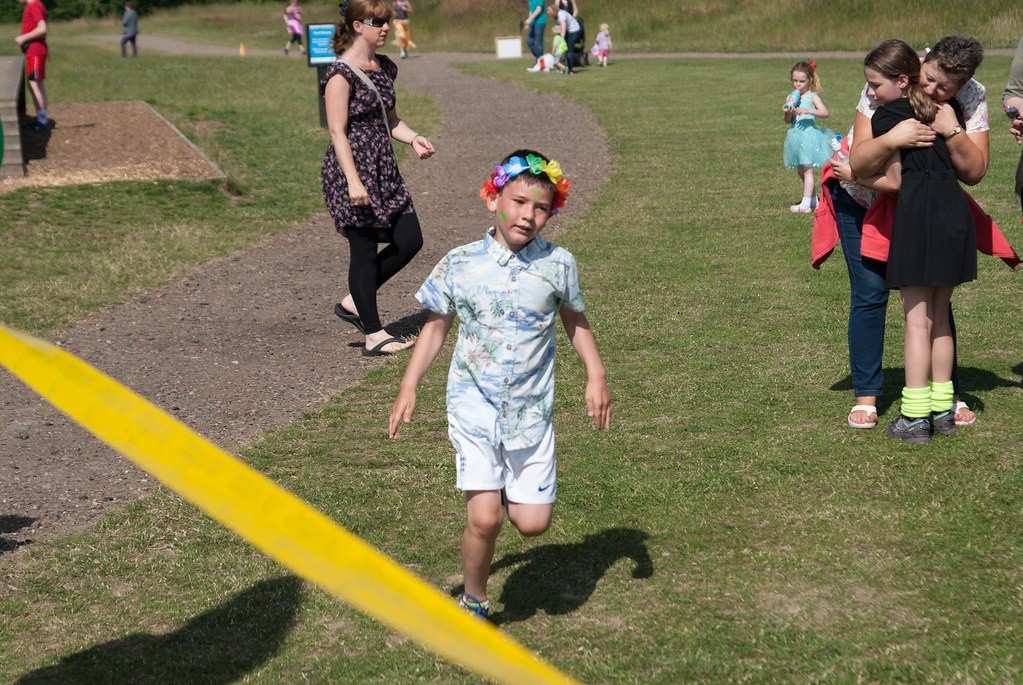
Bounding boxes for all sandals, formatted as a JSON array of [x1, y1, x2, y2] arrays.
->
[[790, 199, 819, 214]]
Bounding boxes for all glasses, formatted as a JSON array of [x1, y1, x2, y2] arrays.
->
[[356, 17, 391, 27]]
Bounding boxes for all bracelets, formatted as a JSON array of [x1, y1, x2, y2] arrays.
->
[[411, 134, 418, 147], [853, 176, 857, 186]]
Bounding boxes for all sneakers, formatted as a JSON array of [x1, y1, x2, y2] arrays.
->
[[886, 414, 934, 443], [932, 410, 958, 436]]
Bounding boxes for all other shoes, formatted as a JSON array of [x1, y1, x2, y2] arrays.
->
[[555, 67, 568, 74], [460, 593, 489, 621], [27, 120, 47, 132]]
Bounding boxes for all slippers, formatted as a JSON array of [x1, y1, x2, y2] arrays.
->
[[848, 402, 877, 429], [362, 334, 414, 357], [335, 301, 367, 335], [951, 402, 977, 425]]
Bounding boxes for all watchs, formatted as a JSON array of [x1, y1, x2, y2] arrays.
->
[[944, 125, 962, 139]]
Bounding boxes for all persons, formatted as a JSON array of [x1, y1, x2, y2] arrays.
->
[[547, 4, 580, 74], [320, 0, 435, 356], [555, 0, 578, 25], [551, 25, 568, 74], [392, 0, 416, 58], [783, 62, 837, 214], [1003, 34, 1023, 146], [524, 0, 546, 58], [282, 0, 306, 55], [829, 36, 989, 428], [15, 0, 52, 132], [119, 3, 138, 57], [596, 23, 612, 67], [829, 39, 977, 443], [390, 148, 611, 621]]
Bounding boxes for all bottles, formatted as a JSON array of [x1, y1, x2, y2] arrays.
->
[[832, 138, 857, 189], [784, 88, 801, 110]]
